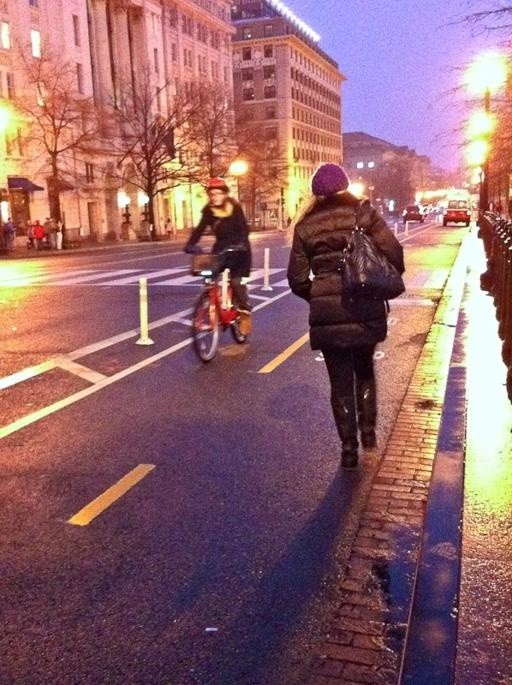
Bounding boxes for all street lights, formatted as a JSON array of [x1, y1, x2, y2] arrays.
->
[[464, 49, 507, 212], [123, 197, 133, 225], [141, 195, 150, 222], [355, 160, 374, 205], [229, 159, 249, 202]]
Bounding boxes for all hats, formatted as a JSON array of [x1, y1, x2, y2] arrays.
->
[[312, 163, 349, 195], [206, 176, 229, 192]]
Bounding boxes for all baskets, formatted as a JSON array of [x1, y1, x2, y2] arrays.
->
[[191, 252, 223, 278]]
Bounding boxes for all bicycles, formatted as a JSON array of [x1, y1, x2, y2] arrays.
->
[[188, 248, 253, 362]]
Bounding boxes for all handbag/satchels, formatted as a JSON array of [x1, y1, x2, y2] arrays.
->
[[340, 200, 406, 308]]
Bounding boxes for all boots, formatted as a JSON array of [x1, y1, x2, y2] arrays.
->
[[356, 380, 377, 448], [235, 308, 251, 336], [330, 395, 360, 468]]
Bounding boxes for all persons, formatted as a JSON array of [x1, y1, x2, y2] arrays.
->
[[183, 178, 252, 337], [165, 218, 172, 238], [286, 163, 406, 472], [3, 217, 60, 252]]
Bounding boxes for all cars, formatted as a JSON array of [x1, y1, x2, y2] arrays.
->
[[402, 199, 471, 228]]
